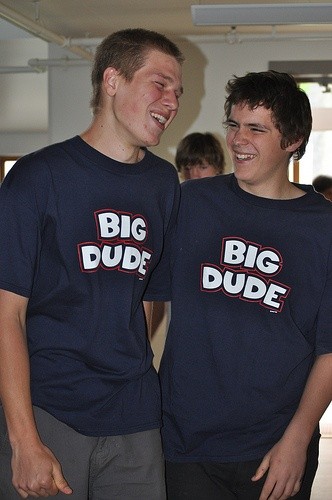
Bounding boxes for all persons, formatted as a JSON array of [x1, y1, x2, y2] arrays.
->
[[1, 28, 184, 500], [312, 175, 331, 201], [157, 70, 332, 500], [174, 131, 227, 181]]
